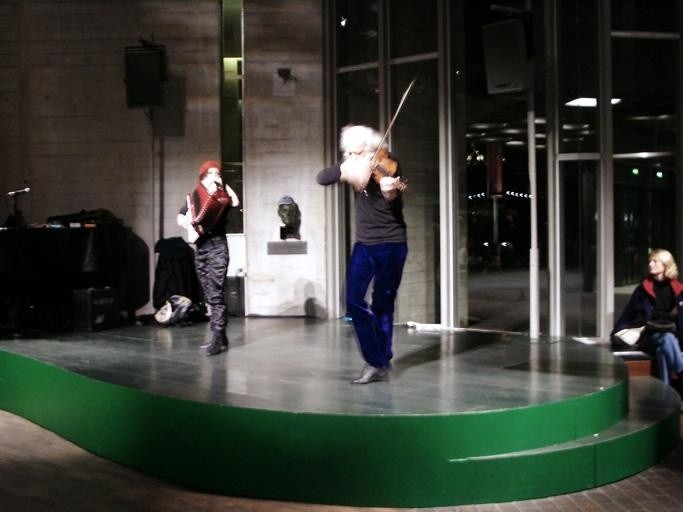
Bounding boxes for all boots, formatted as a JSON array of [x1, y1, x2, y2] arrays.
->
[[199, 327, 229, 356]]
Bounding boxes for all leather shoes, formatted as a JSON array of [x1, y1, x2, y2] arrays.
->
[[351, 359, 393, 384]]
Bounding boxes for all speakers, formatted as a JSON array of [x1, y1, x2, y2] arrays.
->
[[125, 44, 169, 110]]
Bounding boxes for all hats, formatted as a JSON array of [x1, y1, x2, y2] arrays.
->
[[199, 160, 222, 179]]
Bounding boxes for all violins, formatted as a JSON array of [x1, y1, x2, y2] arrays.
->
[[368, 149, 407, 192]]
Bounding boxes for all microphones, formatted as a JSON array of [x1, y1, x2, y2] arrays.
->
[[5, 187, 31, 197]]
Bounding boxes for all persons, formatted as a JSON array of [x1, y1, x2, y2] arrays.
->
[[610, 248, 683, 385], [176, 160, 239, 356], [317, 123, 408, 384]]
